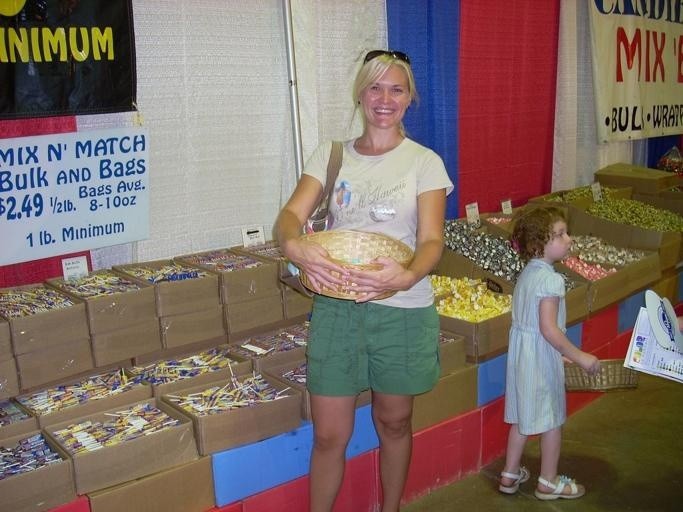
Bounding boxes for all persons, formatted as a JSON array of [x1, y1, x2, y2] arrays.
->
[[271, 50, 456, 512], [498, 207, 600, 501]]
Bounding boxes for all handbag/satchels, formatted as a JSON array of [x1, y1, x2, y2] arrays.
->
[[279, 203, 328, 297]]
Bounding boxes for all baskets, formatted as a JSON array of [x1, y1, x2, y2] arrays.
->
[[299, 230, 414, 301], [564, 359, 639, 391]]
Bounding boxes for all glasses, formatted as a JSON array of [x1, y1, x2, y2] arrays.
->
[[362, 50, 409, 64]]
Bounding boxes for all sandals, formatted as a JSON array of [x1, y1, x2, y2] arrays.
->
[[498, 466, 530, 494], [535, 475, 586, 500]]
